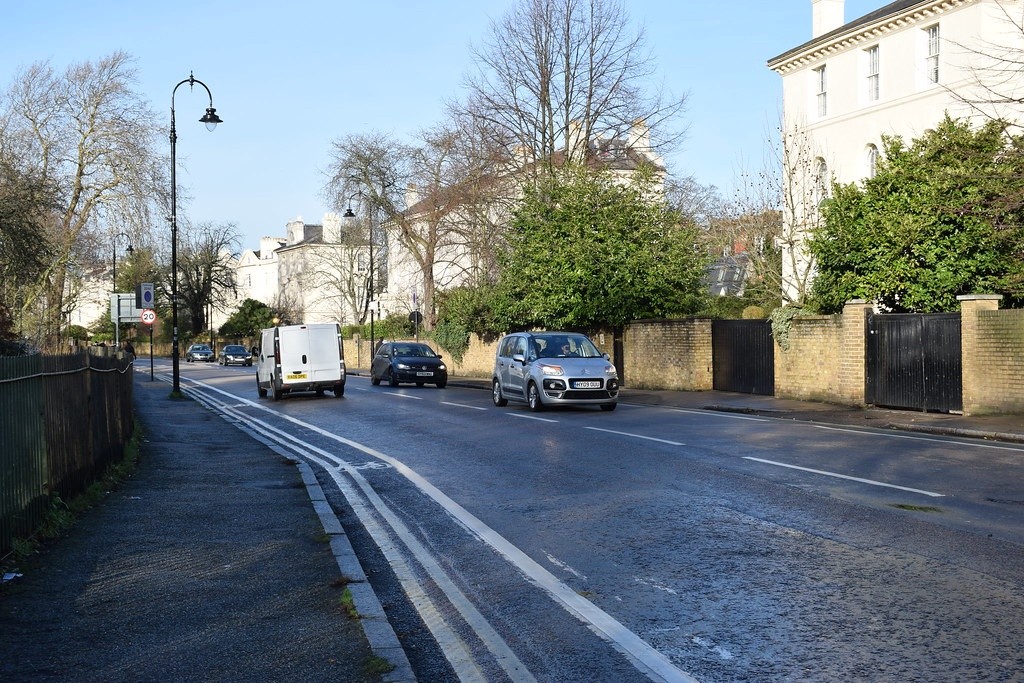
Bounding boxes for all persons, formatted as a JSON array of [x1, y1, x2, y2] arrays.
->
[[413, 348, 421, 356], [558, 342, 578, 355]]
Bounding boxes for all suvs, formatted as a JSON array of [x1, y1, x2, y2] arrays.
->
[[490, 330, 622, 414]]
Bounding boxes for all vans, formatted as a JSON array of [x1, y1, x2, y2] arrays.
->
[[255, 322, 348, 399]]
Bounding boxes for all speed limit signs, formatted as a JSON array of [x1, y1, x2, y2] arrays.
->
[[141, 310, 157, 324]]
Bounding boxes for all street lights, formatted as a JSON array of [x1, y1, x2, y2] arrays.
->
[[170, 70, 223, 399], [113, 233, 134, 293], [343, 189, 375, 360]]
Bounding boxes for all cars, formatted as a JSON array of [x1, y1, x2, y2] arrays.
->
[[185, 343, 216, 363], [369, 343, 447, 389], [219, 345, 253, 367]]
